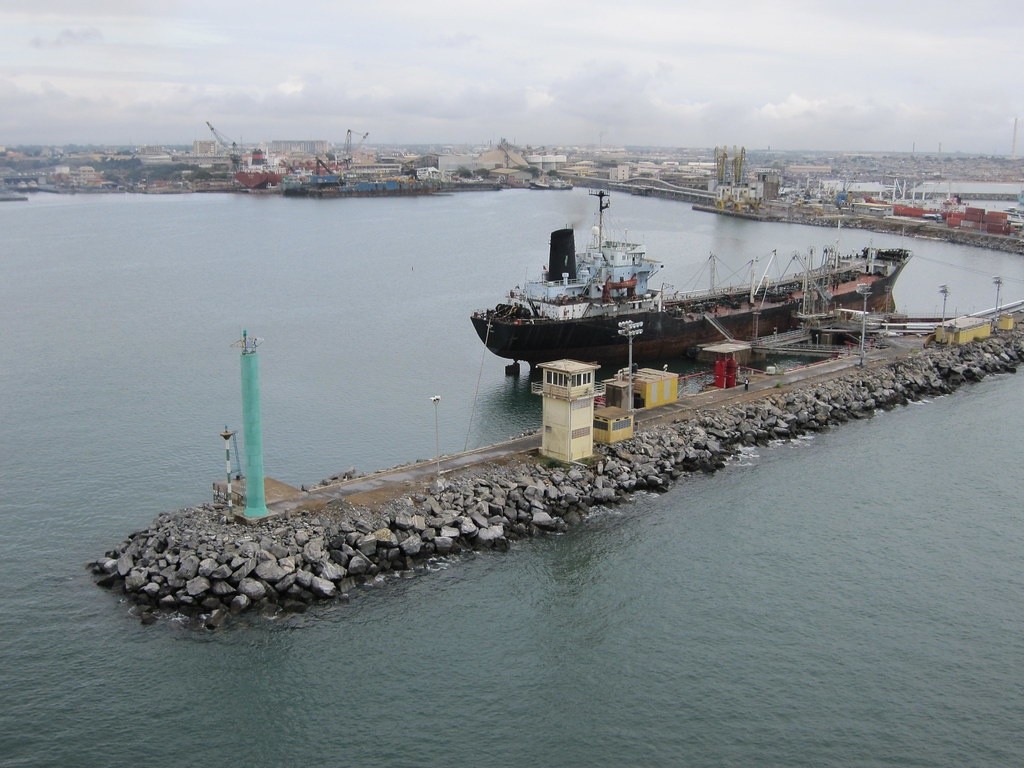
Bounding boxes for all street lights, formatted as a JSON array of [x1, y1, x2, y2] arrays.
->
[[618, 320, 643, 409], [992, 276, 1003, 321], [431, 395, 441, 478], [856, 283, 872, 350], [939, 284, 950, 339]]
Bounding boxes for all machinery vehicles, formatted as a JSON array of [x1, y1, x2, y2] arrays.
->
[[922, 213, 942, 220]]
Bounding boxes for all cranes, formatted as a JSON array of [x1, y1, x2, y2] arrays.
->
[[713, 146, 732, 209], [206, 121, 243, 170], [733, 144, 750, 211], [315, 157, 333, 175], [341, 128, 369, 172]]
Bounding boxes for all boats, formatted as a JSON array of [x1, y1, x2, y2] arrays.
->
[[529, 175, 574, 190], [469, 185, 914, 369]]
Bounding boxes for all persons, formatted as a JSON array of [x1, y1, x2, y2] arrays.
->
[[744, 377, 749, 391]]
